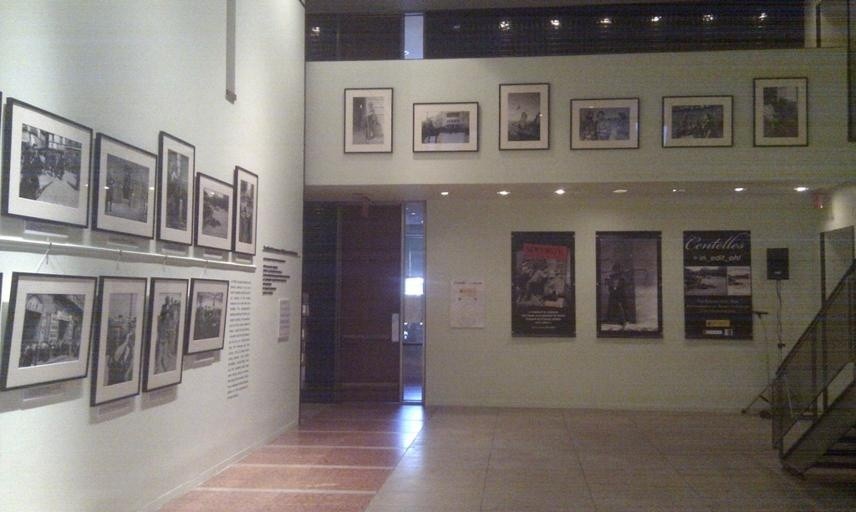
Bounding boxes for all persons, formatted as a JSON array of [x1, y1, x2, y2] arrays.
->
[[523, 266, 550, 301]]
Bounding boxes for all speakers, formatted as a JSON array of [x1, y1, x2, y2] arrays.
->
[[767, 247, 789, 281]]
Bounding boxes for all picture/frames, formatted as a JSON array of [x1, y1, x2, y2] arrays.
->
[[3, 272, 96, 390], [343, 87, 394, 153], [569, 97, 640, 151], [91, 132, 157, 240], [412, 101, 479, 153], [144, 278, 189, 392], [90, 275, 148, 405], [595, 230, 664, 339], [498, 83, 550, 151], [753, 77, 809, 148], [682, 229, 753, 340], [662, 95, 733, 148], [0, 98, 93, 227], [235, 166, 256, 253], [511, 230, 576, 338], [185, 278, 229, 353], [194, 172, 234, 252], [157, 129, 196, 245]]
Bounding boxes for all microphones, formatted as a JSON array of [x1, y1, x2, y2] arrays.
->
[[747, 309, 768, 315]]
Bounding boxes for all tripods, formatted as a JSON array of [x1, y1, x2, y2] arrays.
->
[[739, 281, 805, 422]]
